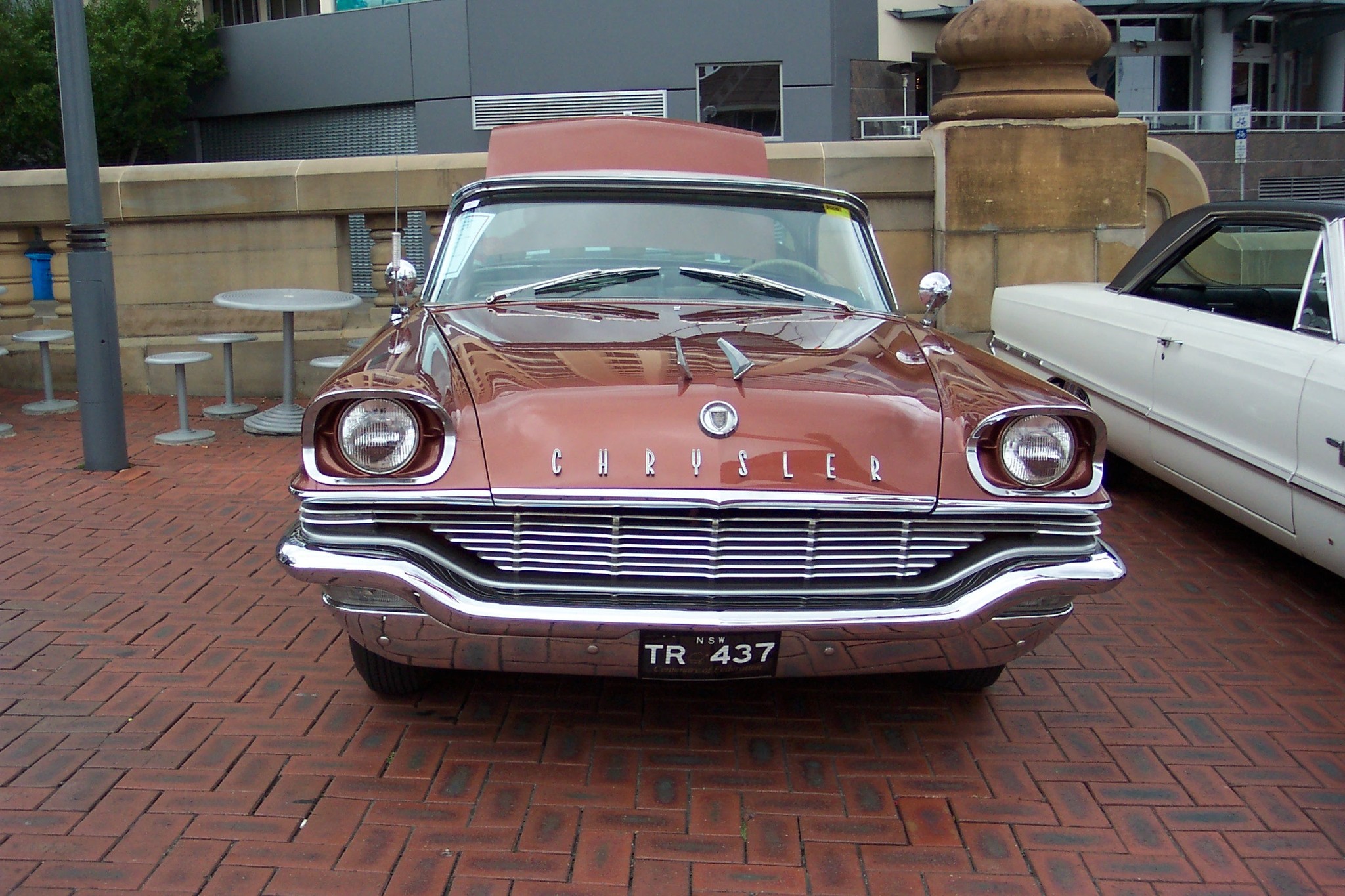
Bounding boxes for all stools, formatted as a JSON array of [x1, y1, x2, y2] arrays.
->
[[343, 338, 368, 348], [12, 330, 78, 415], [195, 333, 260, 420], [309, 356, 350, 369], [144, 350, 219, 446]]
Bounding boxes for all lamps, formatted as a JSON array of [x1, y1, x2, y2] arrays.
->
[[1130, 40, 1147, 48], [1243, 43, 1255, 49]]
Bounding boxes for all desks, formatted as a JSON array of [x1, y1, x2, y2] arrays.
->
[[210, 288, 364, 436]]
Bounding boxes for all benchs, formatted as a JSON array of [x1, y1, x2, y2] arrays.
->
[[1153, 286, 1326, 324]]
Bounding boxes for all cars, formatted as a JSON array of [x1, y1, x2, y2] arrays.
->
[[272, 113, 1127, 697], [987, 199, 1345, 580]]
